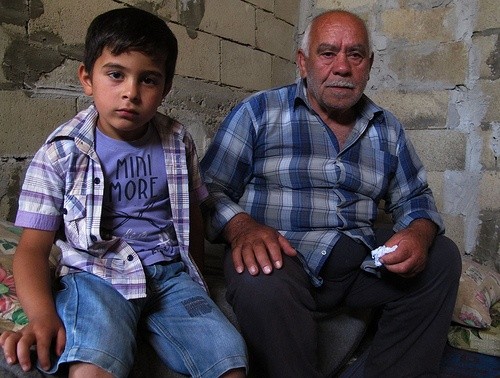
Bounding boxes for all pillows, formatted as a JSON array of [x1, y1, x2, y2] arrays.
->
[[450, 255, 500, 330], [0, 216, 63, 335]]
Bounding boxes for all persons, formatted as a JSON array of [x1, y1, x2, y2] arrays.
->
[[200, 9, 463, 378], [2, 5, 251, 377]]
